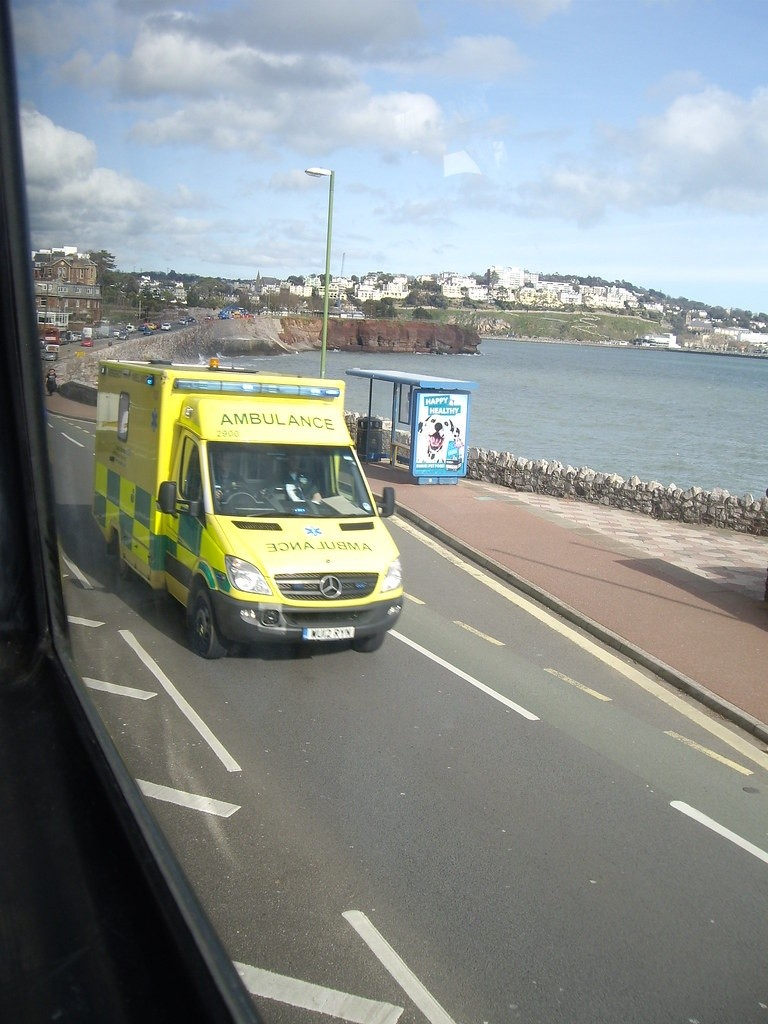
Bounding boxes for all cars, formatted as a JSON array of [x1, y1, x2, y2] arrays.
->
[[44, 352, 58, 361], [176, 318, 188, 324], [71, 334, 78, 342], [126, 323, 137, 333], [74, 331, 85, 341], [138, 324, 147, 331], [161, 322, 171, 331], [113, 328, 130, 336], [118, 331, 129, 340], [144, 329, 156, 335], [187, 316, 195, 322], [145, 323, 158, 329], [81, 337, 93, 347]]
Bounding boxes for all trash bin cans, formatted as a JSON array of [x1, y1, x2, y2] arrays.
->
[[357, 417, 383, 465]]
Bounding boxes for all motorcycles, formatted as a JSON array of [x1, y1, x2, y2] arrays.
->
[[45, 368, 57, 396]]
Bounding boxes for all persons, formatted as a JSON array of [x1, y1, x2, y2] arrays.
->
[[282, 452, 323, 503], [46, 369, 57, 379], [216, 451, 247, 497]]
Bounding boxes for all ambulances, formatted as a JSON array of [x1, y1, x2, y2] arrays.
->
[[91, 357, 405, 659]]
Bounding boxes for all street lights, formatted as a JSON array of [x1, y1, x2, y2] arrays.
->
[[304, 167, 336, 379]]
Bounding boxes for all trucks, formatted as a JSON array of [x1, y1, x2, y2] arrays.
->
[[44, 328, 67, 345]]
